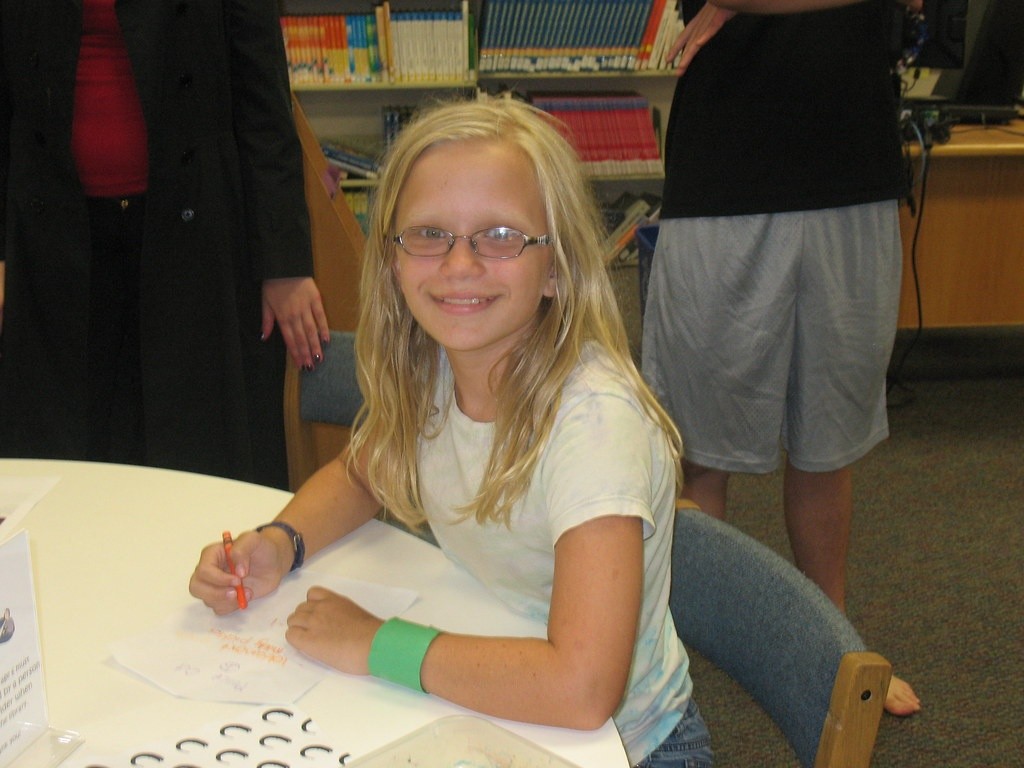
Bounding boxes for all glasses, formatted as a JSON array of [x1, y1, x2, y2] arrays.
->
[[385, 225, 550, 259]]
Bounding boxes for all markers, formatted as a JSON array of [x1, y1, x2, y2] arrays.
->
[[220, 530, 247, 611]]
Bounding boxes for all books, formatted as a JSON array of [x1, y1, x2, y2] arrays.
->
[[531, 90, 664, 176], [322, 104, 419, 237], [598, 191, 663, 264], [479, 1, 686, 73], [280, 11, 463, 85]]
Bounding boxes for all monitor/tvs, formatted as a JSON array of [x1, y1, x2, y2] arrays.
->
[[899, 0, 968, 70]]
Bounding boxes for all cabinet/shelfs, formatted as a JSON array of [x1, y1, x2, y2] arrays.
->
[[277, 0, 687, 355]]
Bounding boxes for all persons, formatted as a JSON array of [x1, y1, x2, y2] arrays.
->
[[639, 0, 925, 713], [189, 98, 714, 768], [0, 0, 331, 492]]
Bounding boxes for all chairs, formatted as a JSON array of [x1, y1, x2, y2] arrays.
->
[[668, 498, 891, 767], [284, 330, 368, 492]]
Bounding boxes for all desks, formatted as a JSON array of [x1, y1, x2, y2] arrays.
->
[[0, 457, 631, 768], [895, 120, 1024, 329]]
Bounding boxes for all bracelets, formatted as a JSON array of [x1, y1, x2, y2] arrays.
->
[[368, 616, 441, 695], [257, 522, 305, 572]]
